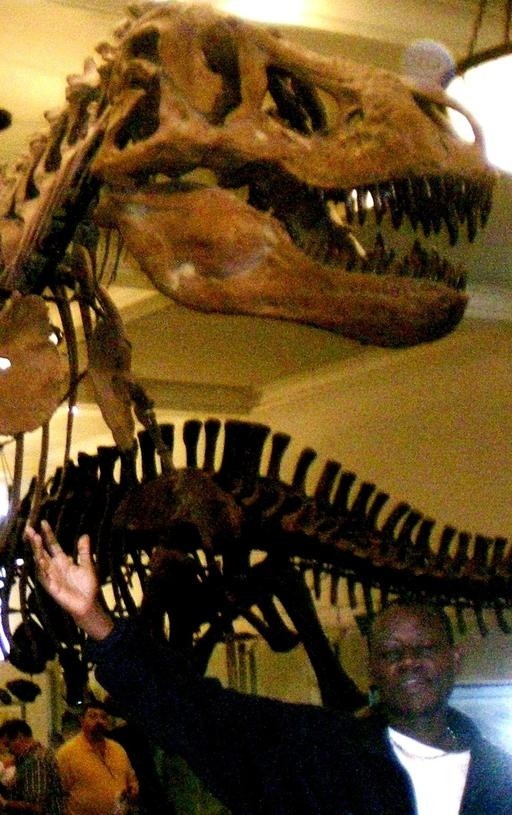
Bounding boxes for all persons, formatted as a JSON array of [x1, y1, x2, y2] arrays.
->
[[1, 718, 64, 815], [25, 516, 511, 815], [50, 700, 139, 813]]
